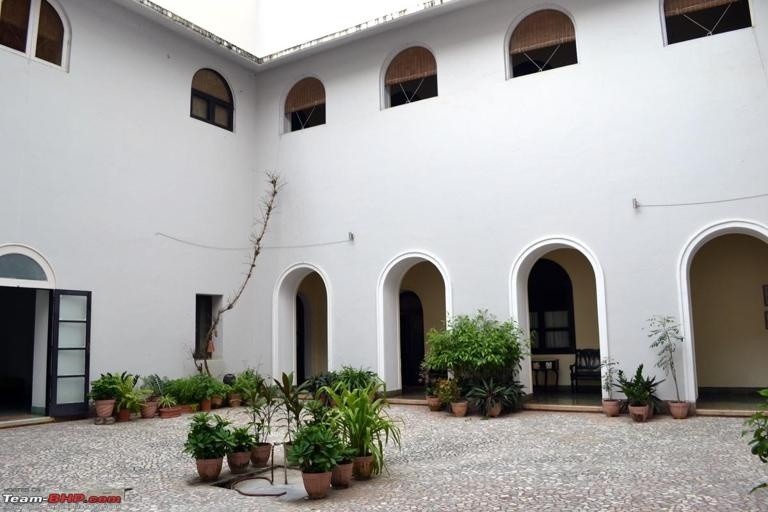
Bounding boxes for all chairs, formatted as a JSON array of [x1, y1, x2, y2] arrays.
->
[[570, 348, 601, 392]]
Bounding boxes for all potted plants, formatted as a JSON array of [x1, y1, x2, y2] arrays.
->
[[86, 363, 408, 499], [638, 314, 690, 419], [600, 359, 663, 422], [419, 310, 530, 418]]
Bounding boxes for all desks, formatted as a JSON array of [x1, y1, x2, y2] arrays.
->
[[532, 359, 559, 394]]
